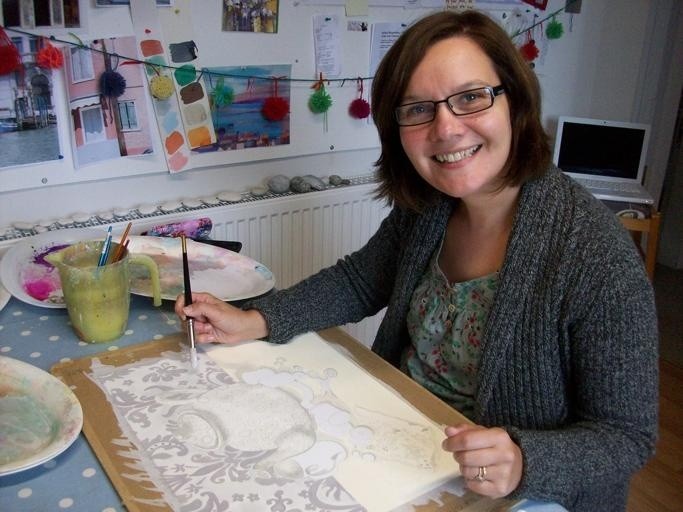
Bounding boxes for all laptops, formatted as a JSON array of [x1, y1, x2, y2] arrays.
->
[[552, 115, 655, 205]]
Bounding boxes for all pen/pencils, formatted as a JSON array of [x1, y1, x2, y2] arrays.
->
[[98, 222, 133, 267]]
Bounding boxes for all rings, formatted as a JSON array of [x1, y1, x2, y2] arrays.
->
[[476, 465, 488, 482]]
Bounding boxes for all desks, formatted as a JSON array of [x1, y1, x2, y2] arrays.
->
[[0, 295, 572, 512], [601, 199, 660, 286]]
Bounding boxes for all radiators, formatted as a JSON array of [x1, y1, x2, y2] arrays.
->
[[0, 173, 395, 352]]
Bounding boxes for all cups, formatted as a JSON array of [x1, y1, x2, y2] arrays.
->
[[273, 440, 347, 482]]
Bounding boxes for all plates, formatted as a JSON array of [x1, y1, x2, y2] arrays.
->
[[0, 355, 83, 476], [112, 235, 276, 303], [0, 230, 114, 309]]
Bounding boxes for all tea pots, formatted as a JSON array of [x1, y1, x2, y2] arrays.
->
[[173, 370, 317, 478], [42, 240, 164, 343]]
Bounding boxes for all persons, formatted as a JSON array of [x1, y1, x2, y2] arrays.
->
[[169, 8, 663, 512]]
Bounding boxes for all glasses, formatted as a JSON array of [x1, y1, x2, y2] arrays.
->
[[394, 83, 508, 129]]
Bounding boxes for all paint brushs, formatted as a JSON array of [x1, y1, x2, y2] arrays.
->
[[181, 233, 198, 370]]
